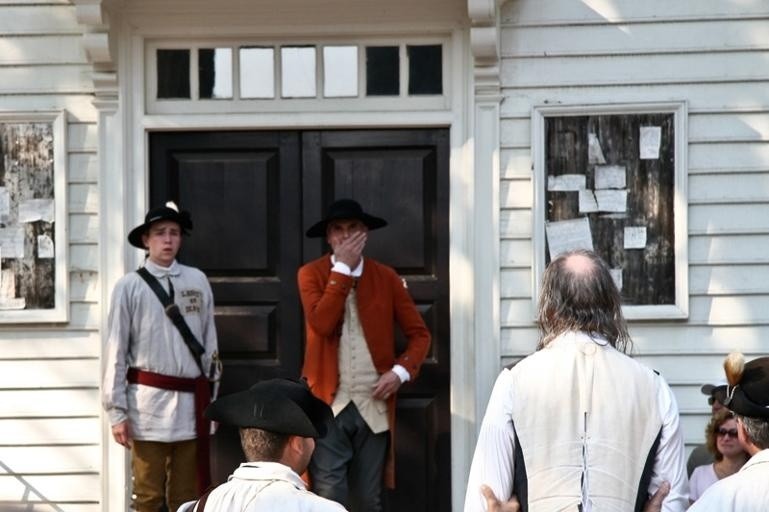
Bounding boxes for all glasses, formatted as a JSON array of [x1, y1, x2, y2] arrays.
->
[[717, 428, 738, 440]]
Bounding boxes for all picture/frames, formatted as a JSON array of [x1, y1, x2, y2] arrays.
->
[[531, 99, 690, 322], [0, 109, 71, 323]]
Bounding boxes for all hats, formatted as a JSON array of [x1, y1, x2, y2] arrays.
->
[[712, 359, 769, 421], [306, 198, 388, 238], [206, 379, 335, 439], [127, 205, 190, 250]]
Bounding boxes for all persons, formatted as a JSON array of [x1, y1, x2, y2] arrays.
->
[[464, 248, 690, 512], [643, 353, 768, 512], [297, 198, 432, 512], [175, 378, 520, 512], [100, 199, 223, 511]]
